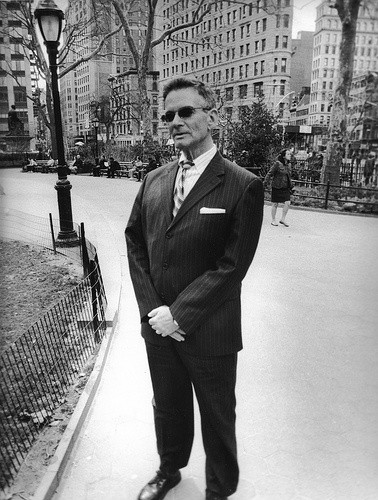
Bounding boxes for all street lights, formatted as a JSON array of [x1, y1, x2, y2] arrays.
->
[[33, 0, 82, 248]]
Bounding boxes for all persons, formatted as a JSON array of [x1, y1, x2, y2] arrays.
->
[[6, 105, 25, 136], [27, 158, 37, 173], [69, 156, 77, 170], [299, 155, 378, 185], [92, 155, 157, 181], [72, 155, 82, 175], [125, 78, 265, 500], [262, 149, 290, 227], [41, 156, 55, 173]]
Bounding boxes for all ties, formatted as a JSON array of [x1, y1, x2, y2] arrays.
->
[[173, 160, 195, 217]]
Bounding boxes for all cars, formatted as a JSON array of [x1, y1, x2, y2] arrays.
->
[[93, 161, 161, 181], [23, 158, 77, 175], [75, 160, 95, 174]]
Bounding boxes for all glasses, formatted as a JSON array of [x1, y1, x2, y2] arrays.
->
[[164, 106, 211, 123]]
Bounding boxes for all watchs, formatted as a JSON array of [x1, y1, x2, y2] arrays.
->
[[173, 318, 179, 325]]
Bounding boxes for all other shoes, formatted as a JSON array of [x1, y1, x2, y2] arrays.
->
[[279, 220, 289, 227], [271, 222, 277, 226]]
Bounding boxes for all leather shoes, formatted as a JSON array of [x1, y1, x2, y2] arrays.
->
[[140, 469, 181, 500], [205, 489, 227, 500]]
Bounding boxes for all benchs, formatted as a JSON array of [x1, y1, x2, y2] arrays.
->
[[33, 159, 60, 173], [98, 162, 160, 178]]
